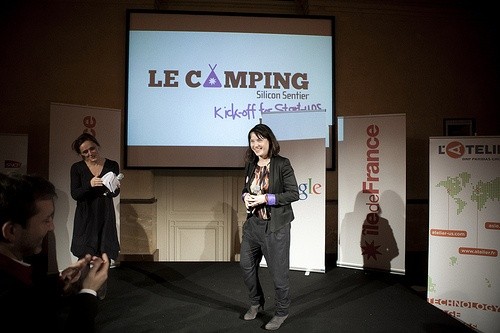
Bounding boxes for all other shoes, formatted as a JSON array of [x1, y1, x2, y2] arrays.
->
[[98, 283, 107, 301]]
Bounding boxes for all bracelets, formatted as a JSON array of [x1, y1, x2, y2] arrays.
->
[[265, 193, 270, 202]]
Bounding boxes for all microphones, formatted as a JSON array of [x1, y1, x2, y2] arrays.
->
[[247, 185, 260, 217], [103, 173, 124, 196]]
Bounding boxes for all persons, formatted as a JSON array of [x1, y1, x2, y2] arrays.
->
[[70, 132, 124, 301], [240, 123, 300, 330], [0, 172, 109, 333]]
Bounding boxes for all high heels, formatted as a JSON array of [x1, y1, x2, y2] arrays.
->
[[265, 314, 289, 330], [243, 302, 264, 320]]
